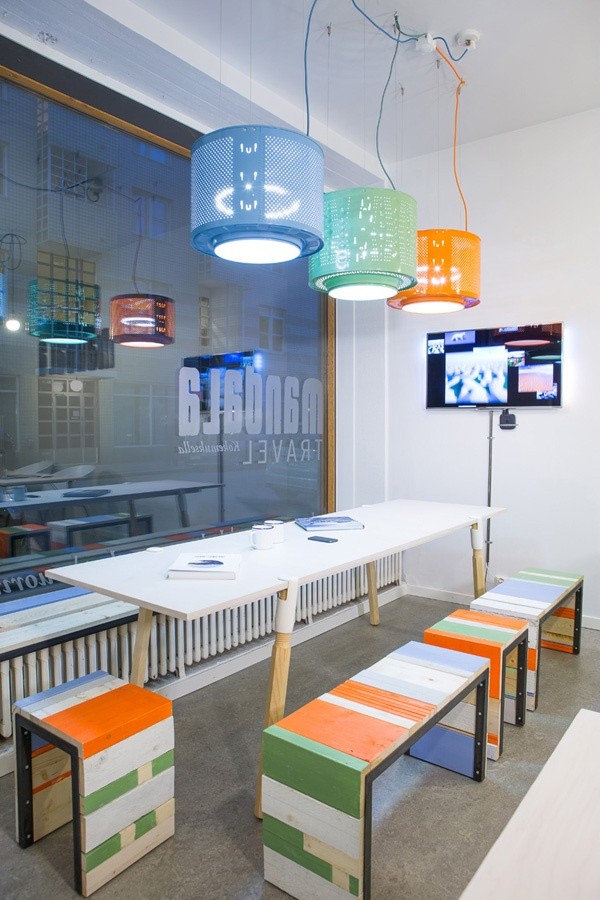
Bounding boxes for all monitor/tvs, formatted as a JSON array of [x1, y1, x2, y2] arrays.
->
[[427, 323, 563, 409]]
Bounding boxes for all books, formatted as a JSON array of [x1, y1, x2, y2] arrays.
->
[[166, 553, 241, 580], [61, 488, 112, 497], [295, 516, 365, 532]]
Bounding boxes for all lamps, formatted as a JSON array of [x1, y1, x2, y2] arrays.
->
[[26, 168, 102, 344], [385, 32, 481, 315], [192, 0, 325, 264], [109, 187, 175, 349], [308, 0, 418, 301]]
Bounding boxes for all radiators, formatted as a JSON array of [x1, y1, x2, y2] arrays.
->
[[0, 550, 409, 778]]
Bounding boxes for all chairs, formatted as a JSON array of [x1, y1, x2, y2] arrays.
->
[[12, 457, 96, 485]]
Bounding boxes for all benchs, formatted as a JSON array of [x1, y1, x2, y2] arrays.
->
[[47, 512, 153, 547], [262, 565, 584, 900], [0, 524, 51, 560]]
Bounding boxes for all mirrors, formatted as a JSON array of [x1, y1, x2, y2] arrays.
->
[[0, 65, 336, 615]]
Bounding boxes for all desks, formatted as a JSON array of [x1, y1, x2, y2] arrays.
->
[[0, 479, 226, 537], [45, 501, 508, 821], [2, 473, 77, 492]]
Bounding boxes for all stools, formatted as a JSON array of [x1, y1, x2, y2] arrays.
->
[[12, 670, 175, 898]]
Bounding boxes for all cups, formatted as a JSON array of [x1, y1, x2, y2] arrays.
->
[[0, 486, 15, 501], [14, 485, 27, 501], [264, 520, 287, 543], [249, 525, 275, 549]]
